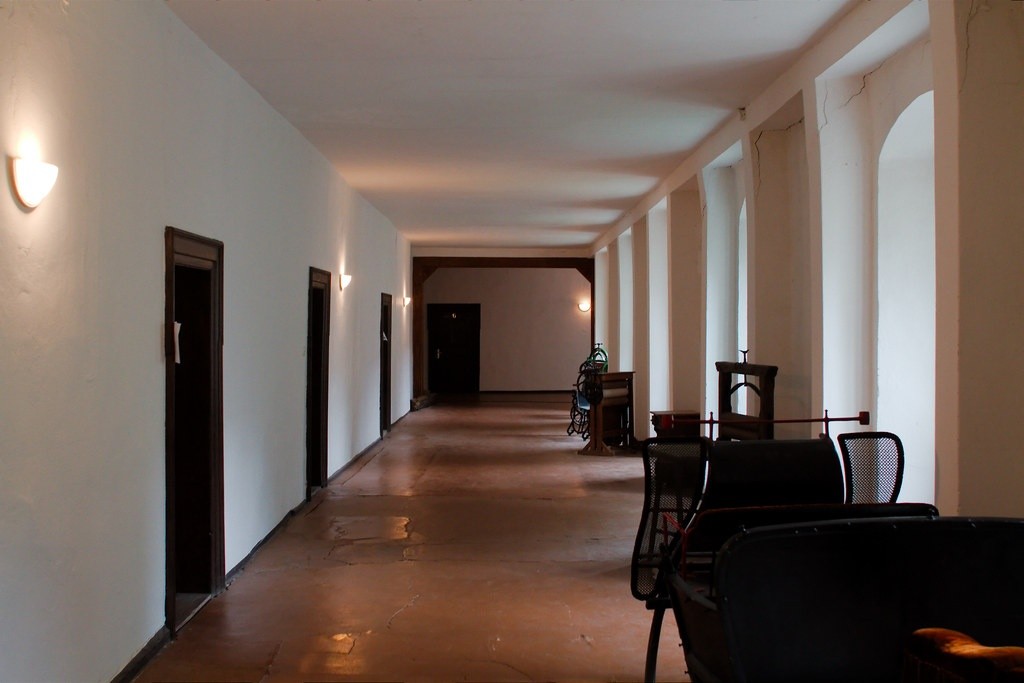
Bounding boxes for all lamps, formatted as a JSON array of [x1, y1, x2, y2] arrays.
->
[[8, 156, 59, 210], [339, 274, 351, 290], [578, 303, 591, 311], [403, 297, 410, 306]]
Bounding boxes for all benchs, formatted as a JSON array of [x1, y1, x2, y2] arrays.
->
[[631, 429, 1024, 683]]
